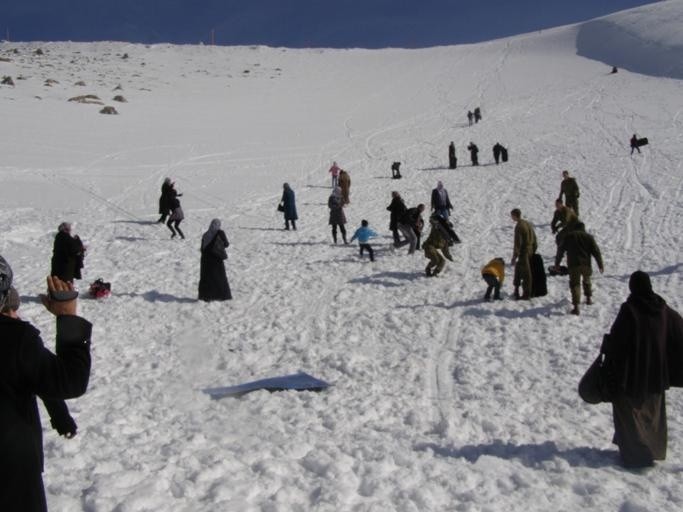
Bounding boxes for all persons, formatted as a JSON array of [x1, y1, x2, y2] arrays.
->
[[610, 271, 683, 467], [167, 191, 184, 238], [282, 182, 298, 230], [199, 219, 232, 301], [0, 256, 93, 512], [158, 178, 173, 224], [51, 222, 84, 282], [630, 134, 640, 154]]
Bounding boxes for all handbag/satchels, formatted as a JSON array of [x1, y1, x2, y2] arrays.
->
[[277, 201, 286, 212], [211, 234, 228, 261], [577, 351, 611, 405]]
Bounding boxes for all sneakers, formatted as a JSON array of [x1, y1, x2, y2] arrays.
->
[[284, 226, 290, 231], [484, 292, 531, 301], [292, 227, 297, 231]]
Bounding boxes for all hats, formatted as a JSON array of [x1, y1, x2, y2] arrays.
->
[[626, 270, 654, 292]]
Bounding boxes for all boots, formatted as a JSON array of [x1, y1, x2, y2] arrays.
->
[[425, 266, 432, 278], [586, 294, 595, 306], [570, 302, 582, 317], [432, 268, 440, 279]]
